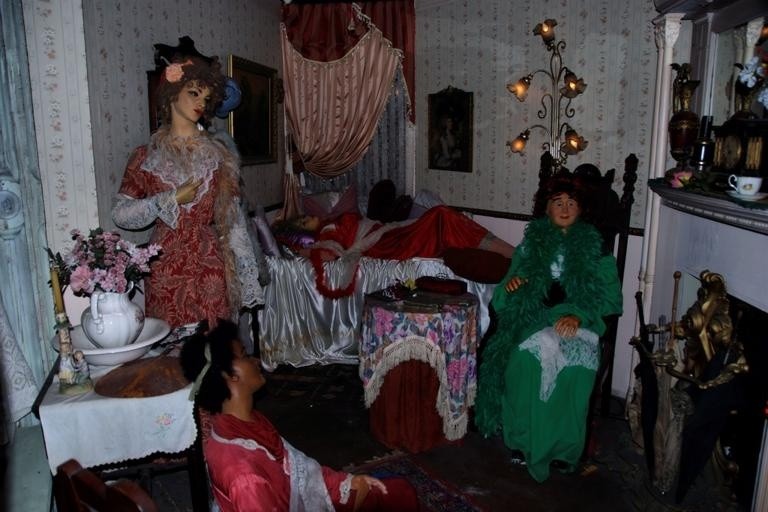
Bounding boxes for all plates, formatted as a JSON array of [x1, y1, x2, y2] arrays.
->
[[725, 190, 768, 202]]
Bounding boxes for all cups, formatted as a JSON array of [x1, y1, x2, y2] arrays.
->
[[727, 174, 763, 196]]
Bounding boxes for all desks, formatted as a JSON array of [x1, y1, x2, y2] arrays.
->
[[357, 290, 479, 456], [32, 340, 210, 512]]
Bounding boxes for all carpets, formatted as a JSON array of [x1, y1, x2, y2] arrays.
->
[[330, 447, 492, 512]]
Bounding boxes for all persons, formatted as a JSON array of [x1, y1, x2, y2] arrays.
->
[[472, 171, 624, 485], [270, 204, 517, 260], [179, 318, 419, 512], [110, 58, 266, 345]]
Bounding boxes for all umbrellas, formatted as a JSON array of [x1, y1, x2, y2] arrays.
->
[[634, 271, 743, 504]]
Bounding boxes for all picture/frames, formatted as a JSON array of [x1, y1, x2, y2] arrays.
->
[[146, 35, 222, 135], [227, 53, 285, 167], [427, 85, 474, 174]]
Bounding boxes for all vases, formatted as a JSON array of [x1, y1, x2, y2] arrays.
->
[[81, 280, 145, 350], [668, 61, 701, 170]]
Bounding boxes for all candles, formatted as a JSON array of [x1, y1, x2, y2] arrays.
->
[[50, 262, 63, 313]]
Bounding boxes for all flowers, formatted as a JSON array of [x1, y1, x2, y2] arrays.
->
[[730, 21, 768, 96], [667, 171, 707, 193], [41, 226, 163, 301]]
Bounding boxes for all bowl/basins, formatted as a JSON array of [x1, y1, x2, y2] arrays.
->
[[49, 317, 172, 367]]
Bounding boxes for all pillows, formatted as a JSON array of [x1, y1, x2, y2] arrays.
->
[[241, 180, 363, 286]]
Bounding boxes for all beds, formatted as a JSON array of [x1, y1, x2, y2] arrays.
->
[[229, 189, 645, 421]]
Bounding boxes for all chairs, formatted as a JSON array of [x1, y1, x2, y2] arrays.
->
[[51, 458, 158, 512], [477, 151, 639, 465]]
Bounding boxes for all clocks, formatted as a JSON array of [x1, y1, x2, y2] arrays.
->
[[709, 111, 767, 176]]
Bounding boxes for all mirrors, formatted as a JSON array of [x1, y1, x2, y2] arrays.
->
[[706, 14, 768, 142]]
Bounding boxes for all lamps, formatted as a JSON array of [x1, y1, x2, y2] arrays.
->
[[505, 18, 588, 175]]
